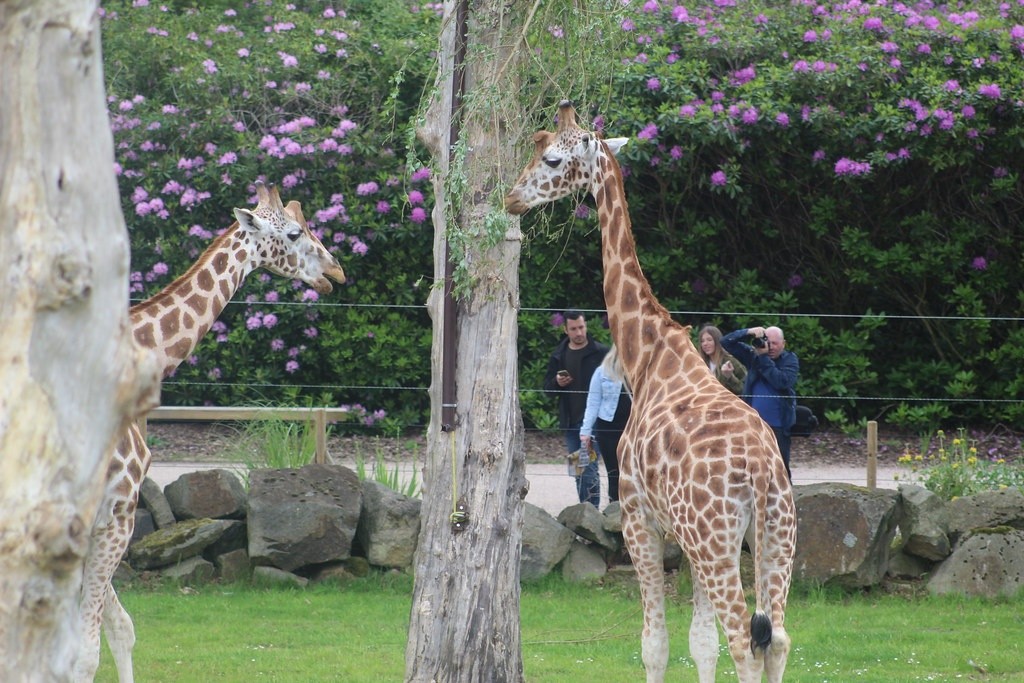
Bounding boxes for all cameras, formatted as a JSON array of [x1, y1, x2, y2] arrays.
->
[[752, 333, 770, 349]]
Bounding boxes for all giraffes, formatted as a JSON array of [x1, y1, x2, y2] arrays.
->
[[69, 182, 346, 683], [506, 102, 796, 683]]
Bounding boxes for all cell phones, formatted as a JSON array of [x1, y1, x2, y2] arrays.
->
[[557, 370, 569, 377]]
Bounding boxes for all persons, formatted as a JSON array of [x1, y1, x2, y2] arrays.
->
[[718, 325, 799, 488], [698, 326, 747, 396], [579, 340, 633, 504], [542, 311, 610, 508]]
